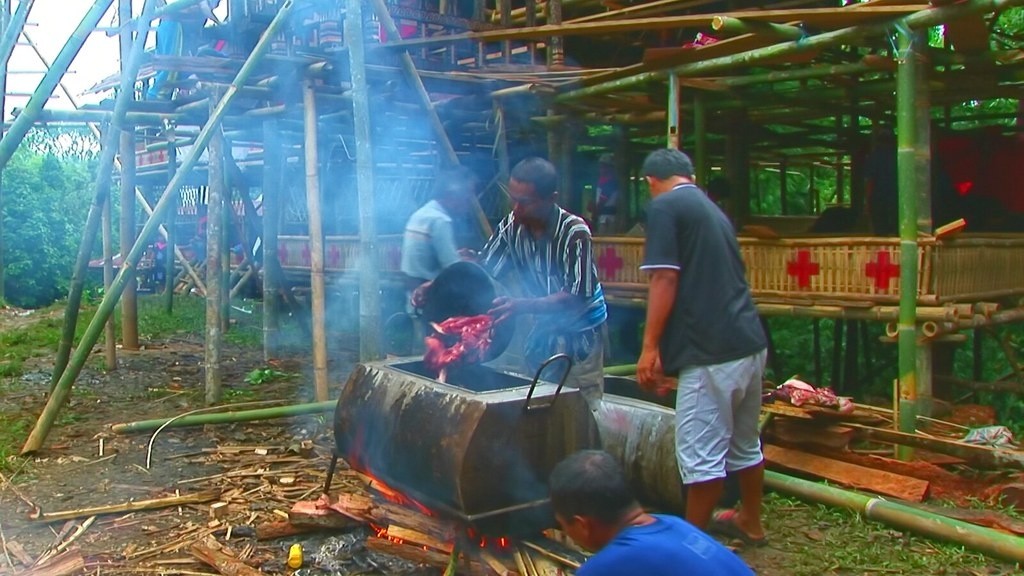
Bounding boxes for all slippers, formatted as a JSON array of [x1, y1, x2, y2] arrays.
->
[[709, 509, 769, 548]]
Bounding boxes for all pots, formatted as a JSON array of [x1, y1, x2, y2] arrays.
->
[[414, 260, 516, 364]]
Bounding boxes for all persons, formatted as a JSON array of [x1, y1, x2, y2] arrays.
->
[[861, 117, 975, 404], [549, 450, 757, 576], [636, 149, 768, 550], [411, 157, 608, 422], [584, 153, 624, 236], [144, 0, 227, 100], [140, 203, 240, 285], [400, 166, 481, 355], [699, 178, 739, 234]]
